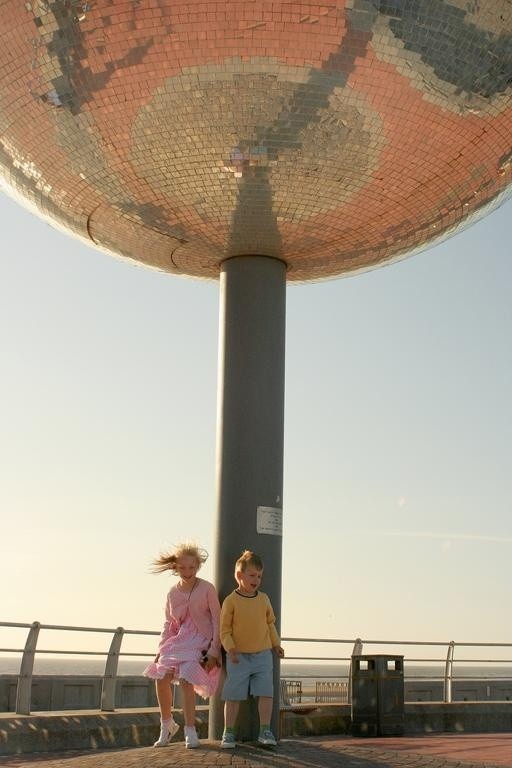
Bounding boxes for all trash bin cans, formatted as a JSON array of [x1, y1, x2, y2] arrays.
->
[[352, 655, 405, 737]]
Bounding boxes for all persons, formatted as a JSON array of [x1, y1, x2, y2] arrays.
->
[[140, 540, 225, 750], [215, 548, 286, 749]]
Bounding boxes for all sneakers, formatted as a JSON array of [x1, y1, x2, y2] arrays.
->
[[220, 731, 236, 749], [153, 720, 180, 746], [183, 725, 200, 749], [257, 730, 278, 746]]
